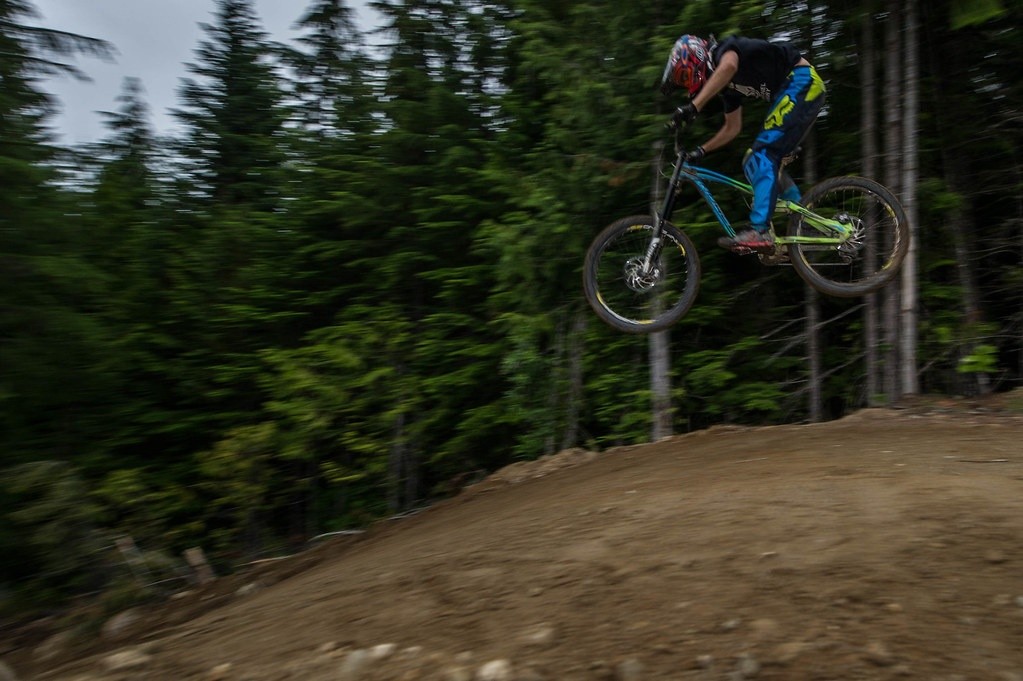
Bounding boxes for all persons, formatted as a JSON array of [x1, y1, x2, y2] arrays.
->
[[661, 36, 827, 252]]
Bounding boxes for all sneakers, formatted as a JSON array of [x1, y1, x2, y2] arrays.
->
[[717, 230, 775, 255]]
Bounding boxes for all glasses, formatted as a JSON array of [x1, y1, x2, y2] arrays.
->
[[676, 67, 692, 89]]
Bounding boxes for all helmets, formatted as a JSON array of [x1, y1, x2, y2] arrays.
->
[[661, 35, 710, 93]]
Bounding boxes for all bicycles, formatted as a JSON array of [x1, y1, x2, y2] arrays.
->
[[581, 107, 911, 334]]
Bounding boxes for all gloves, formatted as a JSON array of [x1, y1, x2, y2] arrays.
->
[[667, 103, 699, 137], [683, 145, 705, 163]]
